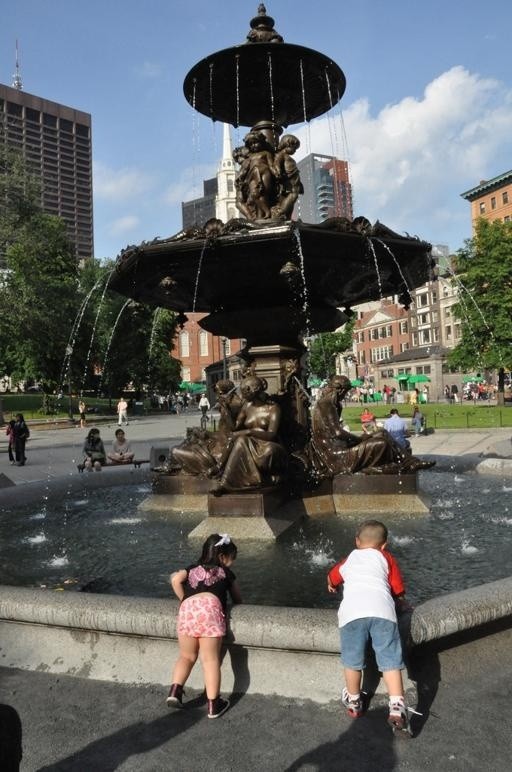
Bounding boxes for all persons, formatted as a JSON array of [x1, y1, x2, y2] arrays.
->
[[242, 131, 276, 221], [13, 414, 30, 466], [270, 134, 304, 223], [462, 381, 496, 401], [117, 397, 129, 426], [423, 385, 428, 404], [411, 406, 424, 438], [107, 429, 134, 464], [166, 533, 242, 720], [159, 393, 190, 416], [82, 428, 106, 472], [451, 383, 458, 403], [344, 384, 392, 405], [198, 393, 210, 422], [208, 375, 291, 497], [310, 375, 437, 475], [444, 385, 450, 399], [327, 519, 423, 739], [6, 420, 16, 465], [231, 147, 252, 222], [149, 378, 242, 478], [78, 400, 87, 428], [384, 408, 412, 450], [361, 408, 376, 434]]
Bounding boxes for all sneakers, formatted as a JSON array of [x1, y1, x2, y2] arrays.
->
[[341, 687, 368, 719], [166, 683, 185, 709], [387, 698, 424, 739], [207, 694, 230, 719]]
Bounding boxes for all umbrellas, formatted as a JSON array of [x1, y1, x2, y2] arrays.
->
[[179, 382, 205, 395], [407, 375, 431, 395], [394, 374, 410, 387], [351, 380, 362, 387], [463, 376, 483, 383]]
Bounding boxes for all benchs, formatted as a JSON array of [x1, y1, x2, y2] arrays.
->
[[375, 415, 427, 436], [76, 459, 150, 471]]
